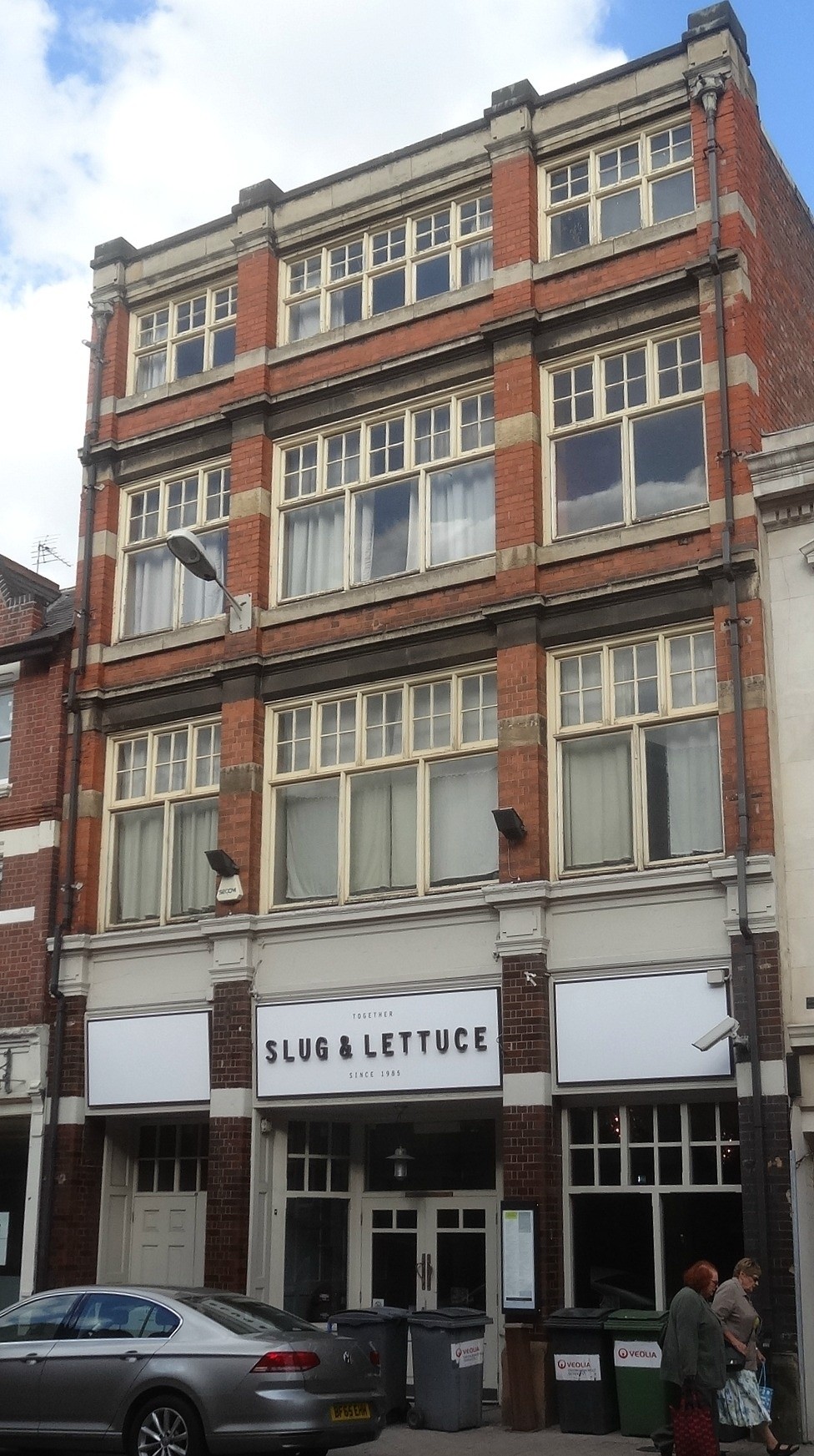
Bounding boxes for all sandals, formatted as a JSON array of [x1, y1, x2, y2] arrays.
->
[[766, 1442, 800, 1456]]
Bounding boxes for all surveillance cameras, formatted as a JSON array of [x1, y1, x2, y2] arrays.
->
[[692, 1017, 740, 1051]]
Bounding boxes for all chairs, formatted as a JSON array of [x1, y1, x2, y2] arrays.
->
[[92, 1307, 134, 1338], [148, 1309, 177, 1338]]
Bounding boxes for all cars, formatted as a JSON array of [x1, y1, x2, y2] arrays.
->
[[0, 1284, 390, 1456]]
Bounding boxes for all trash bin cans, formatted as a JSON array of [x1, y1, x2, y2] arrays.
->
[[407, 1307, 493, 1431], [605, 1309, 670, 1436], [329, 1306, 407, 1425], [543, 1307, 619, 1435]]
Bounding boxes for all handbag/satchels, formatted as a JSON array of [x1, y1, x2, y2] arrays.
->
[[758, 1362, 773, 1415], [725, 1347, 746, 1372], [668, 1385, 720, 1456]]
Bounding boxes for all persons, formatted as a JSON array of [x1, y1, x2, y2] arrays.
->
[[652, 1260, 727, 1456], [712, 1257, 800, 1456]]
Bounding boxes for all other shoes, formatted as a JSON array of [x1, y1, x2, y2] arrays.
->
[[651, 1435, 673, 1456]]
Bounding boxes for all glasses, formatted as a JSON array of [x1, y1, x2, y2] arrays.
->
[[711, 1279, 719, 1286], [751, 1275, 760, 1283]]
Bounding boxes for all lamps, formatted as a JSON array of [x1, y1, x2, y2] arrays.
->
[[167, 528, 254, 633], [201, 851, 242, 878], [384, 1103, 415, 1179], [493, 806, 525, 845]]
